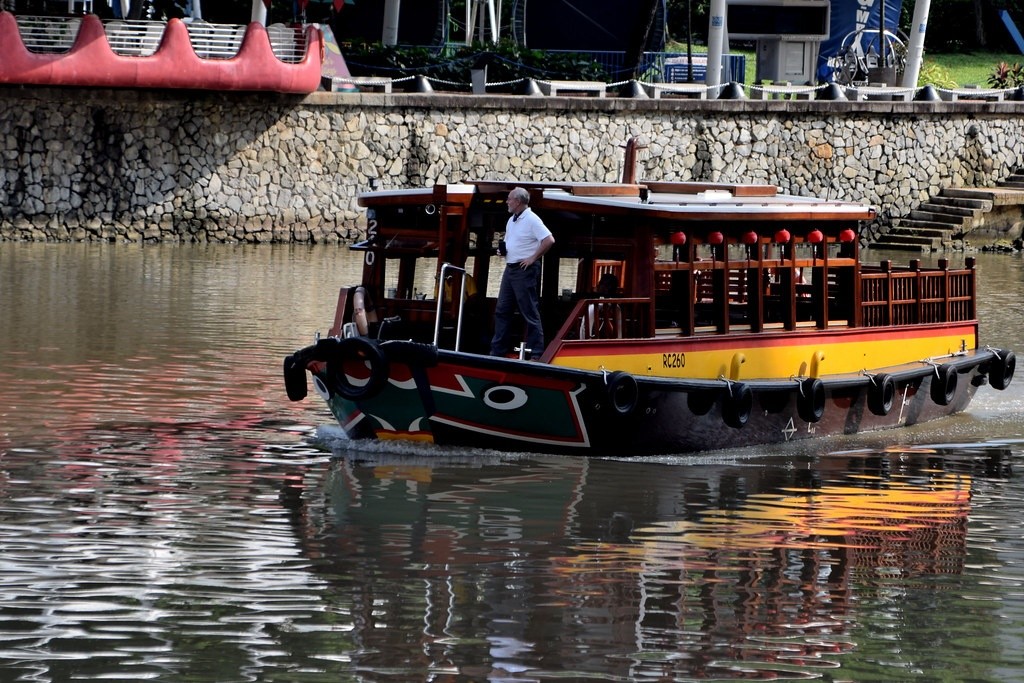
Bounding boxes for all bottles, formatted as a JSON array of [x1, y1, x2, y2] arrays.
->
[[413, 288, 418, 300]]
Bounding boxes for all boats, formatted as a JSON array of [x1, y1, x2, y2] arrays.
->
[[284, 136, 1017, 459]]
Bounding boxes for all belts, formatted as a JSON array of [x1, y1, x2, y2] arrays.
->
[[507, 263, 521, 268]]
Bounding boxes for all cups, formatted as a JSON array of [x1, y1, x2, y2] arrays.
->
[[388, 289, 396, 298]]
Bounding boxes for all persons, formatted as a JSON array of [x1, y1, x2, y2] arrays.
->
[[584, 273, 624, 338], [489, 187, 556, 361], [434, 242, 480, 302]]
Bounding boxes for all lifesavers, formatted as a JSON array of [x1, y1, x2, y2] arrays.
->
[[326, 336, 386, 402], [793, 375, 830, 423], [929, 363, 961, 406], [865, 371, 897, 418], [597, 368, 642, 415], [720, 379, 763, 428], [353, 285, 379, 336], [988, 348, 1017, 390], [282, 355, 309, 402]]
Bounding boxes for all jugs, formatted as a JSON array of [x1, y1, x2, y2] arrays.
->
[[344, 323, 358, 338]]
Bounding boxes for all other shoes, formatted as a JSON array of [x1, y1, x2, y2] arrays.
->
[[530, 356, 541, 362]]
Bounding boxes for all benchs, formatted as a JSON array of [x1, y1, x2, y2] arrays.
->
[[845, 86, 915, 101], [650, 83, 708, 99], [538, 80, 607, 98], [941, 88, 1005, 102], [751, 85, 817, 100], [584, 257, 816, 332], [320, 77, 393, 92]]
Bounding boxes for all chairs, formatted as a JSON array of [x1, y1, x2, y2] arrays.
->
[[55, 18, 297, 63]]
